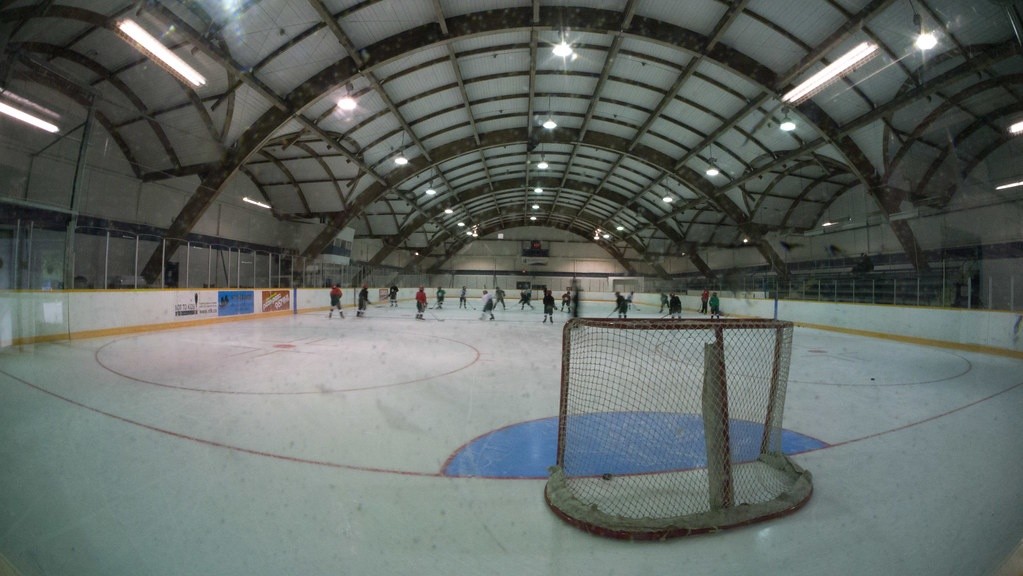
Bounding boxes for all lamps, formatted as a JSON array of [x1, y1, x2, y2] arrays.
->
[[662, 176, 672, 203], [395, 130, 408, 165], [782, 42, 878, 103], [706, 144, 719, 176], [552, 11, 573, 56], [779, 106, 796, 131], [114, 17, 208, 90], [338, 80, 357, 110], [426, 180, 436, 195], [542, 96, 557, 129], [908, 0, 937, 51], [537, 154, 548, 169]]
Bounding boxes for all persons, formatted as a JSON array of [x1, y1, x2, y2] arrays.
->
[[627, 291, 633, 311], [699, 287, 709, 314], [478, 290, 495, 320], [709, 292, 720, 319], [614, 291, 628, 318], [518, 290, 534, 310], [542, 288, 558, 324], [435, 286, 446, 309], [459, 286, 468, 309], [357, 283, 371, 317], [74, 275, 88, 289], [669, 293, 682, 319], [415, 285, 428, 320], [388, 283, 399, 307], [493, 287, 507, 310], [328, 283, 345, 319], [561, 291, 571, 313], [659, 292, 670, 313]]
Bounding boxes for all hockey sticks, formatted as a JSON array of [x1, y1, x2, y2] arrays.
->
[[429, 302, 438, 309], [466, 299, 476, 310], [558, 308, 567, 313], [504, 302, 519, 310], [661, 314, 669, 319], [426, 308, 444, 321], [370, 303, 382, 308], [607, 310, 615, 318], [632, 302, 640, 311]]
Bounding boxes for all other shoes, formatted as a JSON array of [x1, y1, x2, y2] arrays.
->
[[328, 303, 571, 323]]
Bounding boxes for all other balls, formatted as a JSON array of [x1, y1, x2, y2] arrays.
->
[[871, 378, 875, 380], [603, 474, 612, 480]]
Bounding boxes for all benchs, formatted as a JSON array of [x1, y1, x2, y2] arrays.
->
[[688, 265, 960, 307]]
